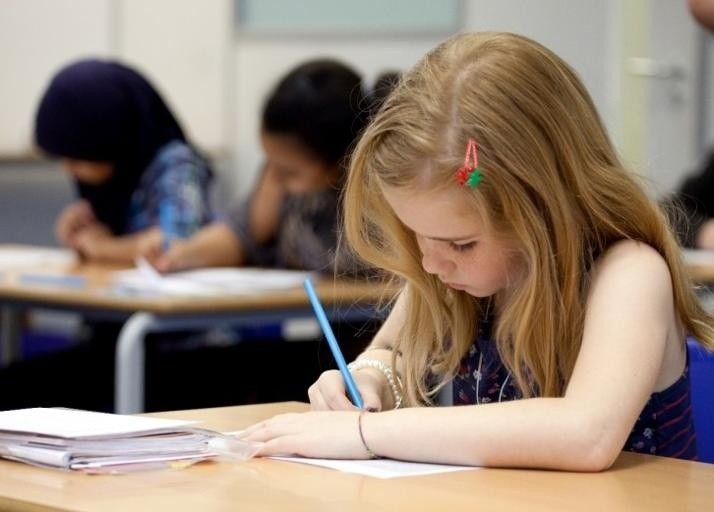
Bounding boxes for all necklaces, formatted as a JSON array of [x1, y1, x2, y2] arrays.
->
[[475, 294, 513, 406]]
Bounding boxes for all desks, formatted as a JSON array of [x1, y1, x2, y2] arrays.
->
[[0, 245, 712, 510]]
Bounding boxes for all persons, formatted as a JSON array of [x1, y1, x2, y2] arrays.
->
[[134, 57, 402, 413], [0, 58, 215, 408], [656, 0, 714, 295], [238, 30, 714, 473]]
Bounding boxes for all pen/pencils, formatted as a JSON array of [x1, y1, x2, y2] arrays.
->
[[301, 279, 366, 409]]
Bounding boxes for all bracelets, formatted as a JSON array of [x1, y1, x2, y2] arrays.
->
[[346, 357, 403, 409], [358, 408, 378, 459]]
